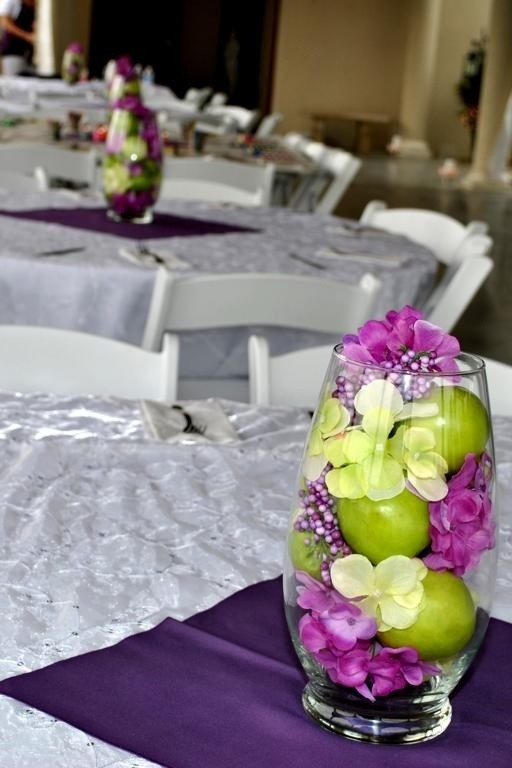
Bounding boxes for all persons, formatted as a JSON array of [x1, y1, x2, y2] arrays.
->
[[0, 0, 38, 77]]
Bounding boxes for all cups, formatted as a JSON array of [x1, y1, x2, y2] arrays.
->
[[63, 43, 149, 110], [97, 102, 161, 229], [279, 339, 504, 749]]
